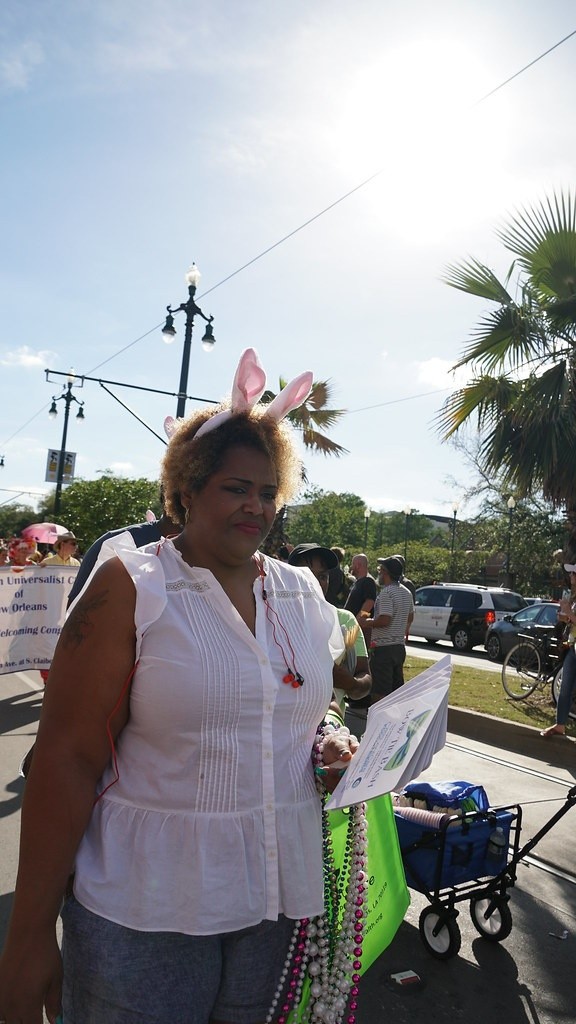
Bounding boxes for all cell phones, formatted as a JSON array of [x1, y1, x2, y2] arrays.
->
[[561, 588, 571, 602]]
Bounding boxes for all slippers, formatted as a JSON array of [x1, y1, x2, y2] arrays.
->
[[540, 728, 566, 737]]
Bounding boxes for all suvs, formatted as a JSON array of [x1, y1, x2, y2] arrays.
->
[[402, 582, 528, 651]]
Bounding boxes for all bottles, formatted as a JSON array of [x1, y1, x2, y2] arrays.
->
[[488, 827, 506, 854]]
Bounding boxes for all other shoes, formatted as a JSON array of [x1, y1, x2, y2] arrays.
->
[[343, 696, 370, 708]]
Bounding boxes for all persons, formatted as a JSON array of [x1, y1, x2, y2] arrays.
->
[[540, 564, 576, 737], [268, 541, 415, 710], [0, 348, 359, 1024]]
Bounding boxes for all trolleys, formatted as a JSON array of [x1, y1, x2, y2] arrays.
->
[[388, 785, 575, 958]]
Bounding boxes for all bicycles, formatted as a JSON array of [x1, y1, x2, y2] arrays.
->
[[503, 624, 575, 721]]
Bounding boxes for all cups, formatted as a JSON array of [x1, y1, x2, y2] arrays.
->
[[392, 806, 448, 829]]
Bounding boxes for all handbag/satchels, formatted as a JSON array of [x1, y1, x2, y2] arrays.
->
[[287, 792, 411, 1024]]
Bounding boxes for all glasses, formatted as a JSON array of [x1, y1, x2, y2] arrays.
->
[[377, 565, 386, 574], [64, 541, 79, 546]]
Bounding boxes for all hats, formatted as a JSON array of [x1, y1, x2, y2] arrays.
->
[[52, 532, 83, 552], [288, 543, 339, 570], [377, 556, 403, 576], [564, 564, 576, 573]]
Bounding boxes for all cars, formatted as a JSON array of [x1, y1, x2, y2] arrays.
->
[[523, 597, 558, 606], [485, 604, 575, 673]]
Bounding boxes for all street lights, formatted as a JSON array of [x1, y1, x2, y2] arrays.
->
[[451, 501, 460, 569], [363, 509, 370, 548], [161, 266, 216, 421], [506, 496, 515, 580], [403, 504, 411, 557], [48, 369, 84, 511]]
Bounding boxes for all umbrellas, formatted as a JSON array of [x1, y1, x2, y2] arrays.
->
[[22, 523, 69, 551]]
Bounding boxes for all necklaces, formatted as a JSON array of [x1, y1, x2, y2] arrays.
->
[[267, 722, 368, 1024]]
